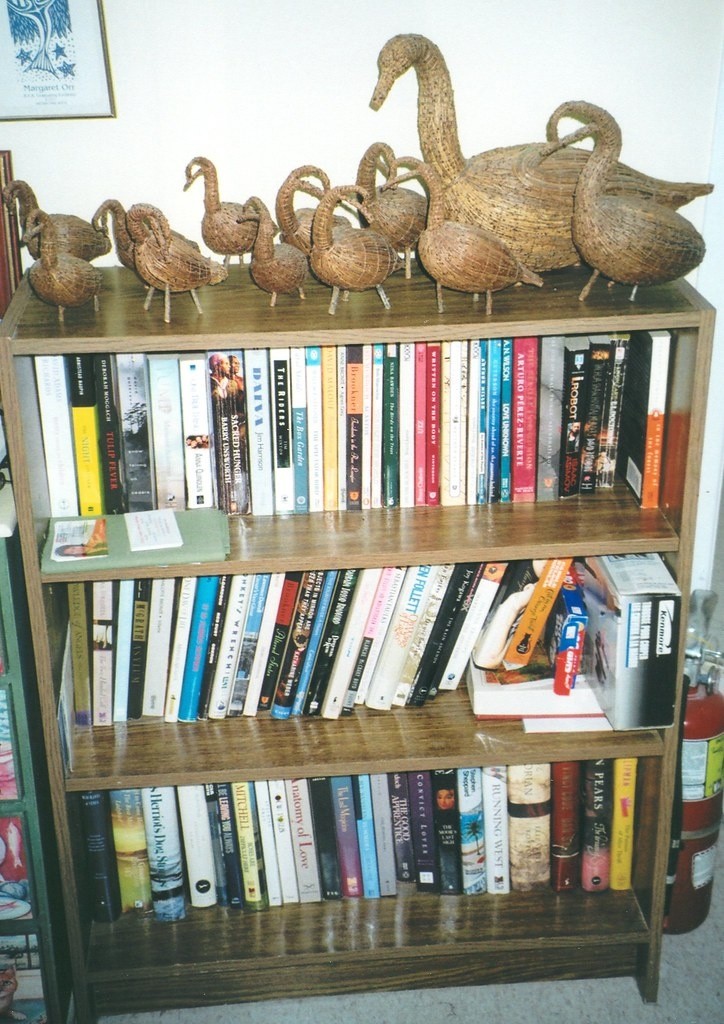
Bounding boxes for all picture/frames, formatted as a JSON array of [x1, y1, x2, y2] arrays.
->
[[0, 0, 117, 122]]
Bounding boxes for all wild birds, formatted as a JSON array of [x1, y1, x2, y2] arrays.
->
[[2, 36, 715, 324]]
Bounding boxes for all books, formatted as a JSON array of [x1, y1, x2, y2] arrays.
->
[[1, 329, 682, 1000]]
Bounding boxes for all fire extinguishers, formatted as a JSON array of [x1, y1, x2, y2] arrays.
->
[[663, 640, 724, 934]]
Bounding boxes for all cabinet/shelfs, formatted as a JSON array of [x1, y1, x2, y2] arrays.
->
[[0, 253, 718, 1024]]
